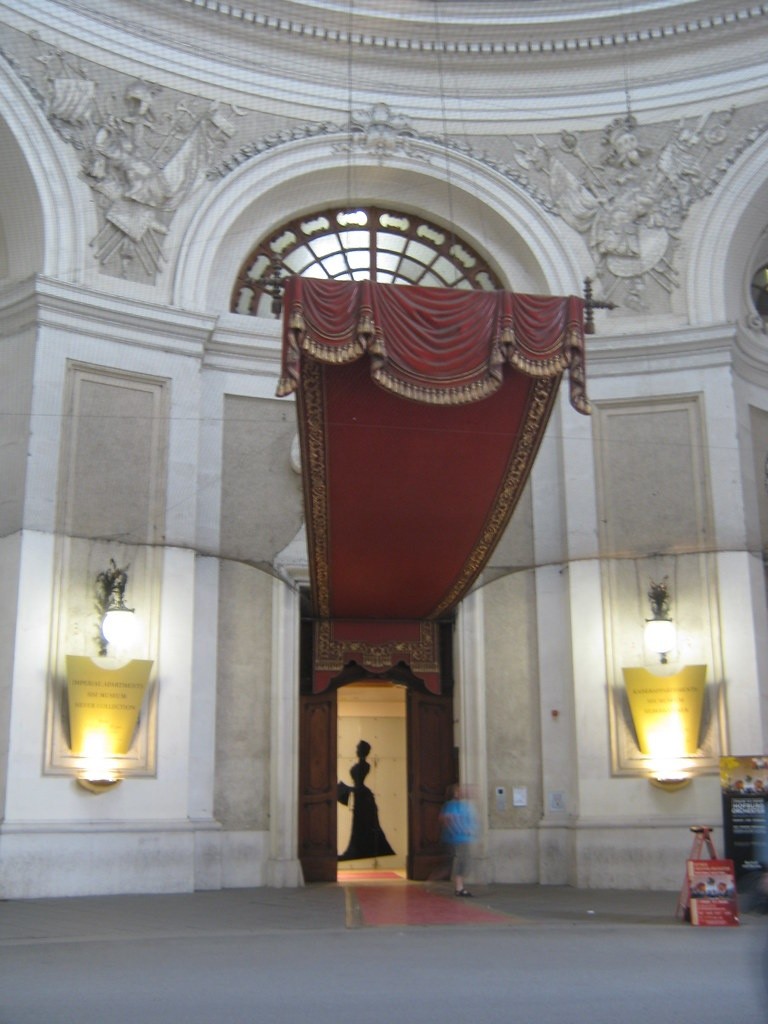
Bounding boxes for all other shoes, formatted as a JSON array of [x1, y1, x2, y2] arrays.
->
[[455, 888, 470, 897]]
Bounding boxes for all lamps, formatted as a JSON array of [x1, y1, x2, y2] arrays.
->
[[94, 559, 137, 657], [648, 772, 692, 794], [644, 583, 676, 664], [76, 776, 124, 793]]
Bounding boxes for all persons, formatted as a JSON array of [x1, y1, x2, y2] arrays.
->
[[733, 776, 765, 795], [437, 780, 487, 898], [694, 877, 731, 897]]
